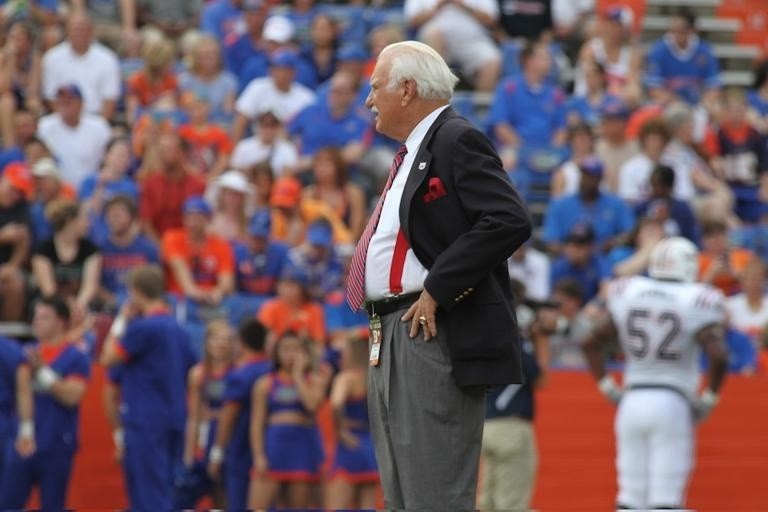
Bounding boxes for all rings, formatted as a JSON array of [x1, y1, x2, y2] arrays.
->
[[419, 316, 427, 325]]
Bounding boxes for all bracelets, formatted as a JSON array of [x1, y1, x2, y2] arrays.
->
[[110, 315, 128, 335], [20, 419, 34, 436], [599, 373, 618, 396], [34, 364, 57, 389], [209, 445, 222, 463], [701, 385, 720, 406], [113, 427, 126, 447]]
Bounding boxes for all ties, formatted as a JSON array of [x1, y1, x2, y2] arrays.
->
[[344, 143, 409, 316]]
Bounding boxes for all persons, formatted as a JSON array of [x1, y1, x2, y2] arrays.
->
[[0, 0, 768, 510], [347, 41, 532, 511]]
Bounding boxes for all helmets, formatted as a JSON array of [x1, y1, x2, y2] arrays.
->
[[645, 235, 701, 282]]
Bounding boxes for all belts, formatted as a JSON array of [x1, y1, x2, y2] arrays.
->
[[365, 291, 425, 319]]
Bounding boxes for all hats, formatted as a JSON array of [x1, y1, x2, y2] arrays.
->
[[605, 5, 636, 30], [271, 53, 295, 69], [261, 17, 292, 42], [576, 157, 604, 178], [560, 222, 593, 246], [8, 163, 34, 200], [219, 170, 247, 194], [307, 224, 329, 247], [270, 179, 303, 207], [596, 94, 630, 119], [248, 214, 269, 238], [184, 199, 210, 217], [30, 157, 53, 175]]
